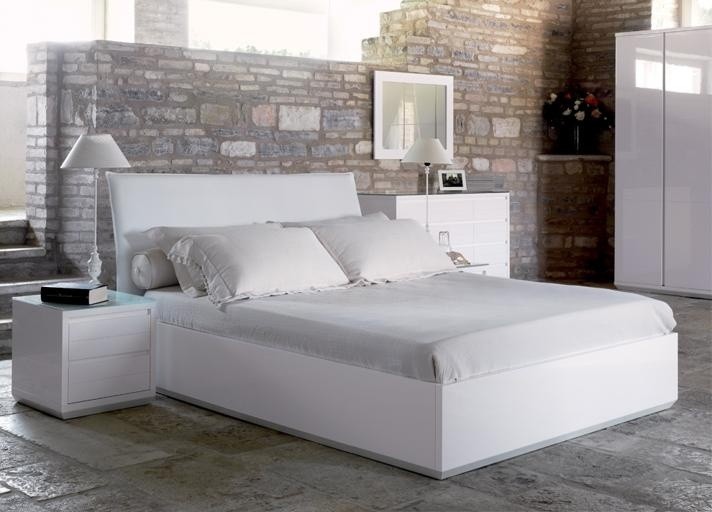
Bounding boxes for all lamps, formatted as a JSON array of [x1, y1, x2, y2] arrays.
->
[[402, 138, 454, 233], [58, 133, 131, 285]]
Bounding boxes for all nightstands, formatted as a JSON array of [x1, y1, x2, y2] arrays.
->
[[12, 289, 158, 420]]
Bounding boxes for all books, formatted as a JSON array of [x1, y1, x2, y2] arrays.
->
[[40, 280, 111, 306]]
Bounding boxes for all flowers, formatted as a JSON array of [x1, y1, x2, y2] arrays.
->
[[541, 82, 615, 134]]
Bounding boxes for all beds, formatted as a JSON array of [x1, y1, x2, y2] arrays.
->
[[103, 172, 677, 481]]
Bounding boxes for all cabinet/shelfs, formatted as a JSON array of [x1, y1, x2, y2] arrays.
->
[[614, 25, 711, 300], [356, 193, 510, 283]]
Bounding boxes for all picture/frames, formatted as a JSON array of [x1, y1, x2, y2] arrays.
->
[[372, 70, 455, 160]]
